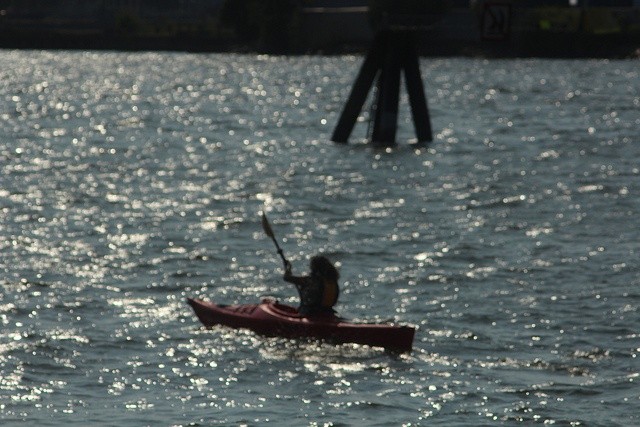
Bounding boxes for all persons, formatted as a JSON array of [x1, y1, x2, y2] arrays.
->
[[283, 256, 342, 322]]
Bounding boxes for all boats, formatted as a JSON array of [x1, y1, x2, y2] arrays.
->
[[188, 298, 420, 352]]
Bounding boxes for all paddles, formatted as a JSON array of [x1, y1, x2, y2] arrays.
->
[[259, 208, 300, 294]]
